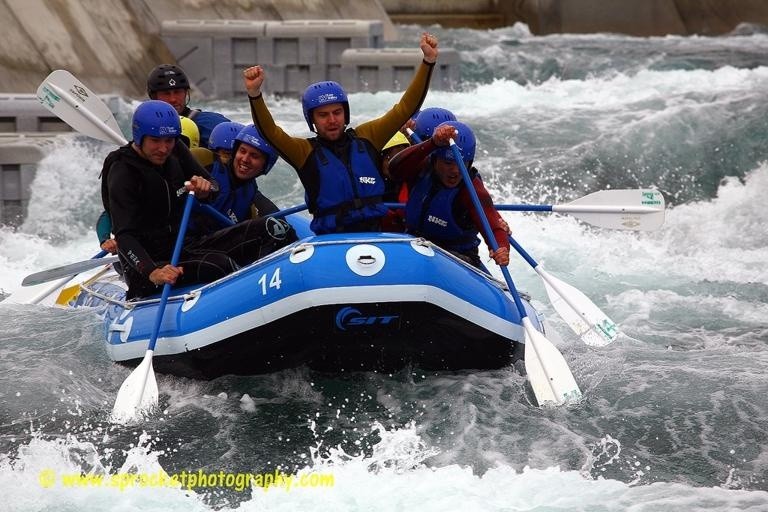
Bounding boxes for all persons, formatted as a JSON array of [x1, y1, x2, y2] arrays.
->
[[95, 61, 295, 294], [382, 108, 516, 273], [244, 32, 438, 237]]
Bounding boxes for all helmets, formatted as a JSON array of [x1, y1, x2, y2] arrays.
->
[[178, 115, 201, 152], [415, 107, 457, 140], [207, 121, 246, 151], [301, 80, 350, 132], [132, 100, 182, 147], [380, 130, 411, 151], [428, 120, 477, 164], [147, 63, 191, 100], [230, 123, 279, 176]]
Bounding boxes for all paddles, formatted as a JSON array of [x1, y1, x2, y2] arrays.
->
[[508, 237, 619, 346], [22, 203, 308, 286], [36, 69, 235, 227], [446, 130, 581, 408], [384, 189, 664, 233], [109, 181, 196, 422]]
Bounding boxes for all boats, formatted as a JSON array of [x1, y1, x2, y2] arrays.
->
[[64, 208, 545, 382]]
